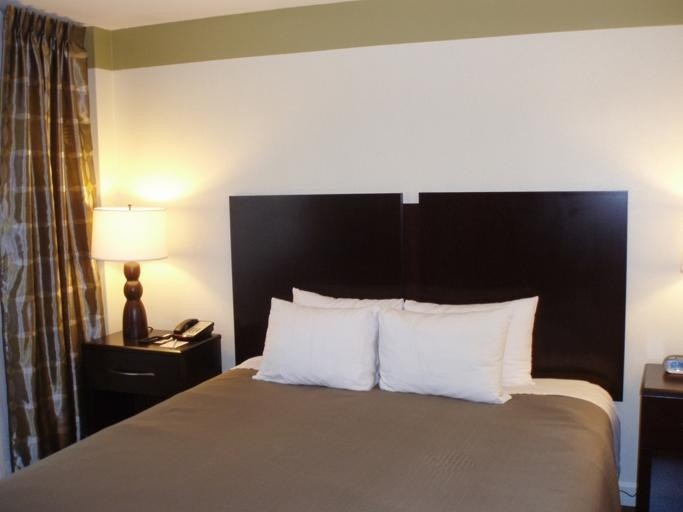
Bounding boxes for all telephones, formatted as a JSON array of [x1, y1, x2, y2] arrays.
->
[[172, 318, 214, 342]]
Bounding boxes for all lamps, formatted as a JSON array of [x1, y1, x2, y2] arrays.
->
[[88, 202, 170, 340]]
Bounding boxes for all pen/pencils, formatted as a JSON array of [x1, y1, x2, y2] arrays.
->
[[173, 339, 177, 347]]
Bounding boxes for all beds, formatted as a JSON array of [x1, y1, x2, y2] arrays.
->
[[1, 186, 631, 511]]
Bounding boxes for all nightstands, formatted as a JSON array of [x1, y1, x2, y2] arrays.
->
[[78, 326, 221, 433]]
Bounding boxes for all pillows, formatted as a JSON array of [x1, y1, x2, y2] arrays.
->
[[250, 285, 539, 406]]
